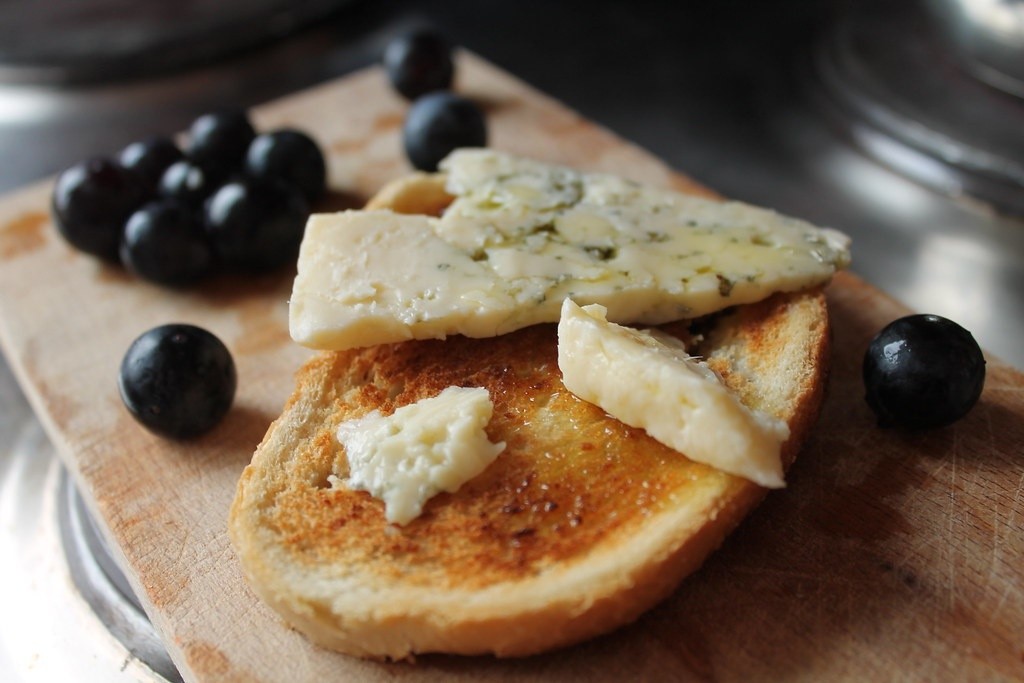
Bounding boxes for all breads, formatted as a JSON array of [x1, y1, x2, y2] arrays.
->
[[232, 171, 836, 660]]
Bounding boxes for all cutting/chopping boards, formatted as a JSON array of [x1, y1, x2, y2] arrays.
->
[[1, 47, 1024, 682]]
